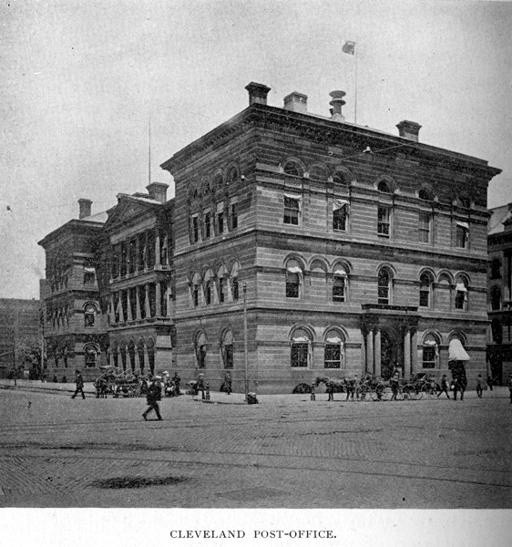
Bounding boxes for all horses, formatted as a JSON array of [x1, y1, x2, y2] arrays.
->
[[314, 376, 353, 402]]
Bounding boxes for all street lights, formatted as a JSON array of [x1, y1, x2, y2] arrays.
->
[[242, 281, 250, 402]]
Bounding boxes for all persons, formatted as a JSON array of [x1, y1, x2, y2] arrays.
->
[[196, 373, 206, 399], [72, 370, 87, 400], [450, 377, 467, 401], [477, 373, 487, 398], [171, 373, 182, 392], [390, 372, 403, 401], [310, 381, 317, 400], [409, 371, 419, 382], [142, 376, 165, 420], [224, 370, 232, 395], [437, 374, 451, 400]]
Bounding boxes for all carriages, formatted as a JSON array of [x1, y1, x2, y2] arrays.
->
[[315, 370, 431, 401]]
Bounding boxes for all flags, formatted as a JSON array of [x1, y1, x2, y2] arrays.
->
[[343, 40, 355, 53]]
